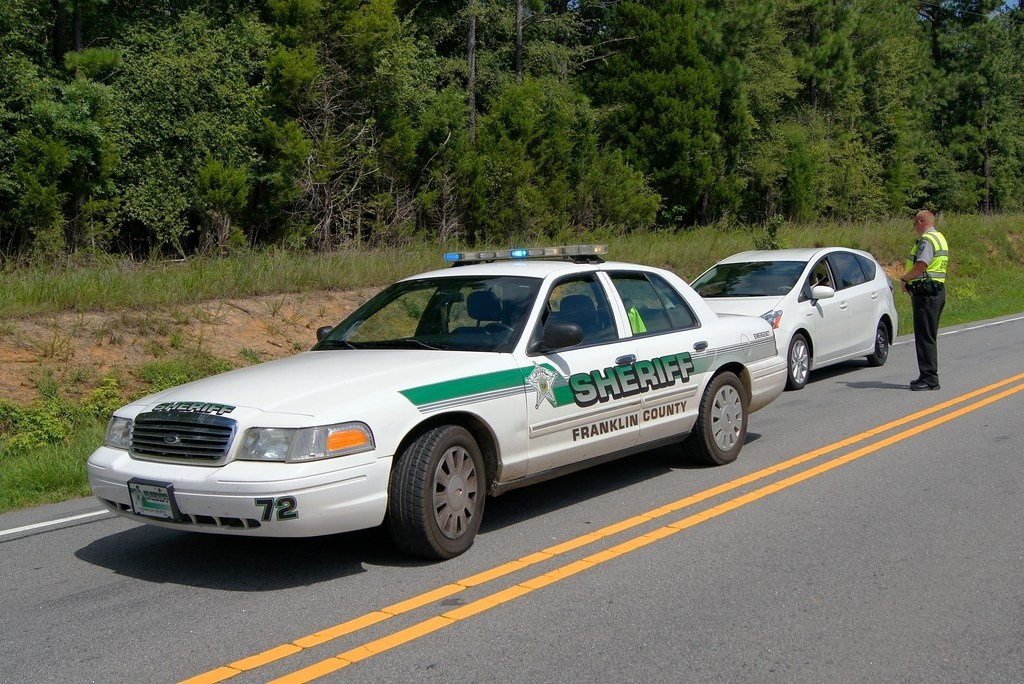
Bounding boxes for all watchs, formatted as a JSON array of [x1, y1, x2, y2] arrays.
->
[[901, 276, 907, 282]]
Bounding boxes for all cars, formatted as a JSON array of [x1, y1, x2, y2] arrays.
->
[[88, 243, 788, 560], [666, 247, 898, 390]]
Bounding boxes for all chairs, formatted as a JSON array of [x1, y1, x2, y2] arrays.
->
[[557, 294, 601, 336], [444, 291, 502, 353], [623, 297, 648, 317]]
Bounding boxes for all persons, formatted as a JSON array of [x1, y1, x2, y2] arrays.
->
[[899, 210, 949, 391]]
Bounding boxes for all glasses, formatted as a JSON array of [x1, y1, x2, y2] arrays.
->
[[913, 221, 917, 226]]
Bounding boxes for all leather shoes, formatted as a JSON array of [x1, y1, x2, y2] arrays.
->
[[910, 382, 941, 390], [910, 379, 919, 385]]
